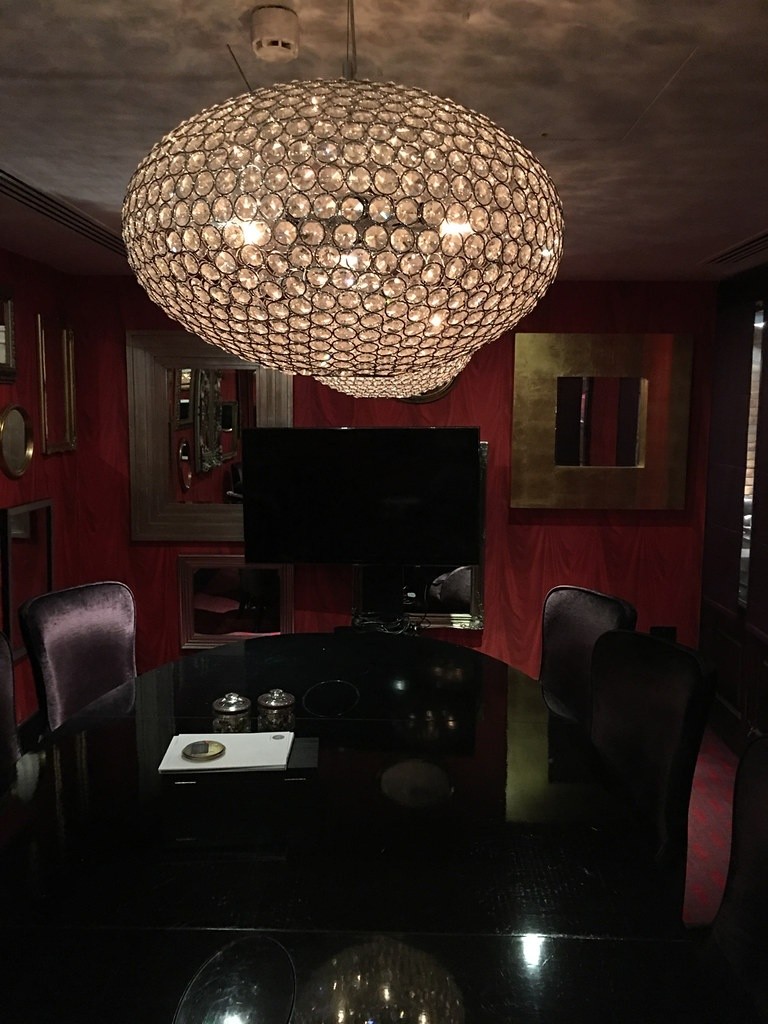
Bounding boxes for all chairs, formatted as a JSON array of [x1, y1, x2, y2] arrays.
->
[[422, 565, 474, 616], [587, 628, 716, 923], [709, 735, 768, 1024], [535, 584, 638, 725], [0, 631, 21, 783], [17, 582, 138, 739]]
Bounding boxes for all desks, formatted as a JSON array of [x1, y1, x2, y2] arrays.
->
[[0, 629, 743, 1024]]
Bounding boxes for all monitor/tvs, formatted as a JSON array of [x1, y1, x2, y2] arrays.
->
[[239, 423, 482, 567]]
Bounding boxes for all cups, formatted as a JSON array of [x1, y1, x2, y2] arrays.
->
[[212, 694, 251, 734], [257, 689, 295, 732]]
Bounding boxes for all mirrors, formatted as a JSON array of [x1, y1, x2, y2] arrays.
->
[[0, 402, 35, 481], [12, 509, 37, 543], [349, 441, 489, 630], [173, 369, 194, 432], [35, 314, 78, 456], [0, 294, 17, 384], [176, 437, 194, 493], [124, 328, 294, 540], [219, 400, 239, 463], [2, 498, 54, 666], [178, 555, 296, 649], [511, 332, 691, 511]]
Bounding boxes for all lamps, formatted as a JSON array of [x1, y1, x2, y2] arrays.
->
[[120, 0, 564, 399]]
